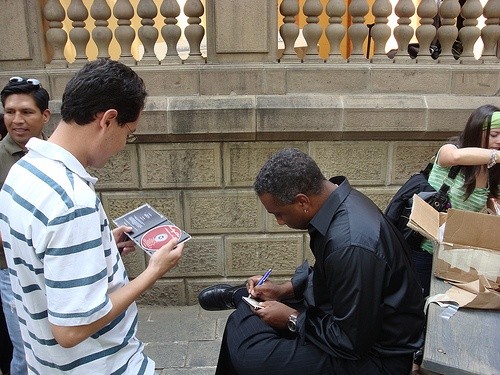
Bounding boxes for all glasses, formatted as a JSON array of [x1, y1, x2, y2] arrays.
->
[[116, 112, 138, 143], [9, 76, 42, 89]]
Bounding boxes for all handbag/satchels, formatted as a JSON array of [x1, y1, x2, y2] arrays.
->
[[384, 144, 461, 255]]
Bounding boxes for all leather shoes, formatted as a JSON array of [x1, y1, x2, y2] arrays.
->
[[198, 283, 248, 311]]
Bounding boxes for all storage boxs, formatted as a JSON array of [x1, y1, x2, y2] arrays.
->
[[406, 193, 500, 292]]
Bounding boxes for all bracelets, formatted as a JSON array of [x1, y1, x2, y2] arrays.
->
[[487, 148, 497, 169]]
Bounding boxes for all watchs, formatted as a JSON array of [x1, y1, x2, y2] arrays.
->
[[287, 311, 301, 334]]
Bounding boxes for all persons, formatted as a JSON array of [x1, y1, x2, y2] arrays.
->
[[0, 57, 184, 375], [199, 146, 425, 375], [0, 76, 51, 375], [387, 0, 466, 59], [411, 104, 500, 375]]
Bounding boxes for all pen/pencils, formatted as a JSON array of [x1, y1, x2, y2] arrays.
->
[[248, 267, 272, 300]]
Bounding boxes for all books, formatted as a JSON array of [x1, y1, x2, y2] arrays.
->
[[241, 296, 263, 309]]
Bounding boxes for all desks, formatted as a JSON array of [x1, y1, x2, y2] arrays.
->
[[420, 254, 500, 375]]
